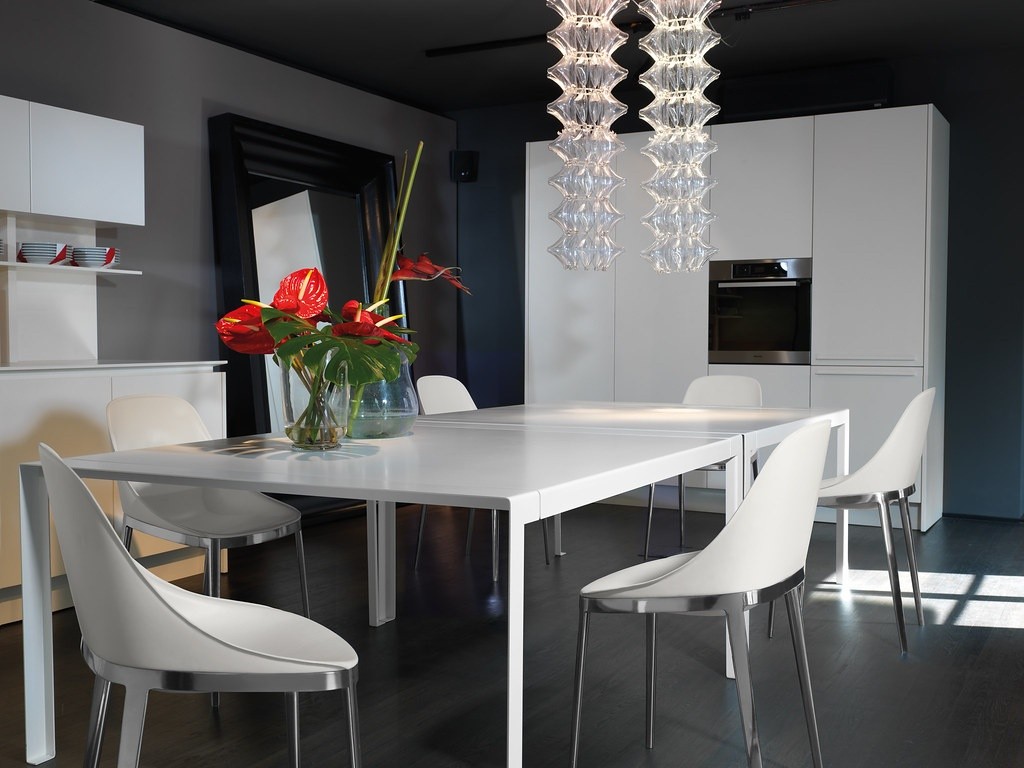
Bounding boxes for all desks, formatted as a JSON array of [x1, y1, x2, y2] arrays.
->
[[19, 402, 854, 768]]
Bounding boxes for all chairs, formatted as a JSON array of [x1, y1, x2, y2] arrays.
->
[[642, 375, 763, 560], [766, 387, 936, 657], [38, 441, 360, 768], [413, 374, 552, 582], [572, 419, 832, 768], [106, 395, 312, 707]]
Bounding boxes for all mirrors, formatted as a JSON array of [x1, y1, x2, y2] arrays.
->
[[207, 110, 415, 515]]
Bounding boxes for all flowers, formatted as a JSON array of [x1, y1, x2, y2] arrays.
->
[[214, 140, 469, 441]]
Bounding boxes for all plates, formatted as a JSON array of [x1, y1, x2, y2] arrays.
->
[[0, 240, 121, 269]]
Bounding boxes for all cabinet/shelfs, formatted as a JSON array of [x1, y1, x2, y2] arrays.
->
[[0, 94, 231, 626], [524, 102, 950, 533]]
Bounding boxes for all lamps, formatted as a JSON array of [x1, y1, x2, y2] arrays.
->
[[546, 0, 722, 275]]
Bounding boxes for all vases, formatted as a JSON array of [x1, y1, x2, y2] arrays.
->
[[328, 301, 420, 438], [274, 347, 352, 448]]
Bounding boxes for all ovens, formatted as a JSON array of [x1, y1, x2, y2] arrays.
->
[[708, 258, 813, 365]]
